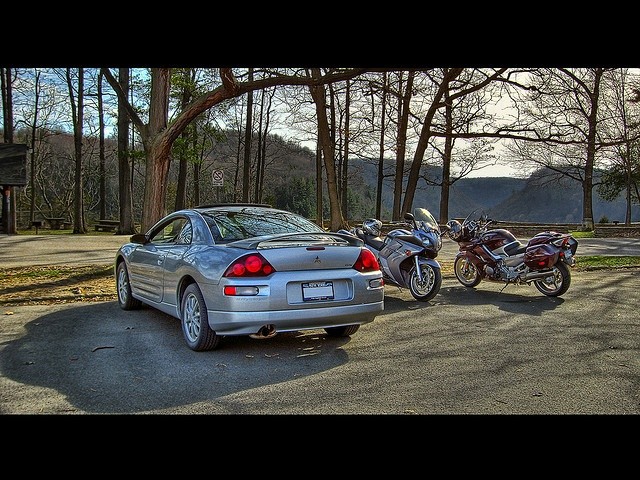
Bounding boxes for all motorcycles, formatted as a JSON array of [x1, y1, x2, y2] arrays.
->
[[335, 208, 450, 302], [446, 209, 578, 297]]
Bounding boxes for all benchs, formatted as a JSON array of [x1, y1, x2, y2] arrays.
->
[[95, 225, 116, 231]]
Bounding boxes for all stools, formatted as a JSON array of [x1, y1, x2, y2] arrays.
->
[[62, 221, 72, 229]]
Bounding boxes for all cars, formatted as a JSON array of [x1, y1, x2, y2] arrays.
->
[[111, 202, 386, 352]]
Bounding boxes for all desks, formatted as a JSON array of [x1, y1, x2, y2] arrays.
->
[[47, 218, 65, 230], [451, 218, 466, 225], [94, 220, 120, 224]]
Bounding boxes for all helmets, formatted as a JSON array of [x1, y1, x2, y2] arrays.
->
[[446, 220, 465, 241], [363, 218, 382, 236]]
